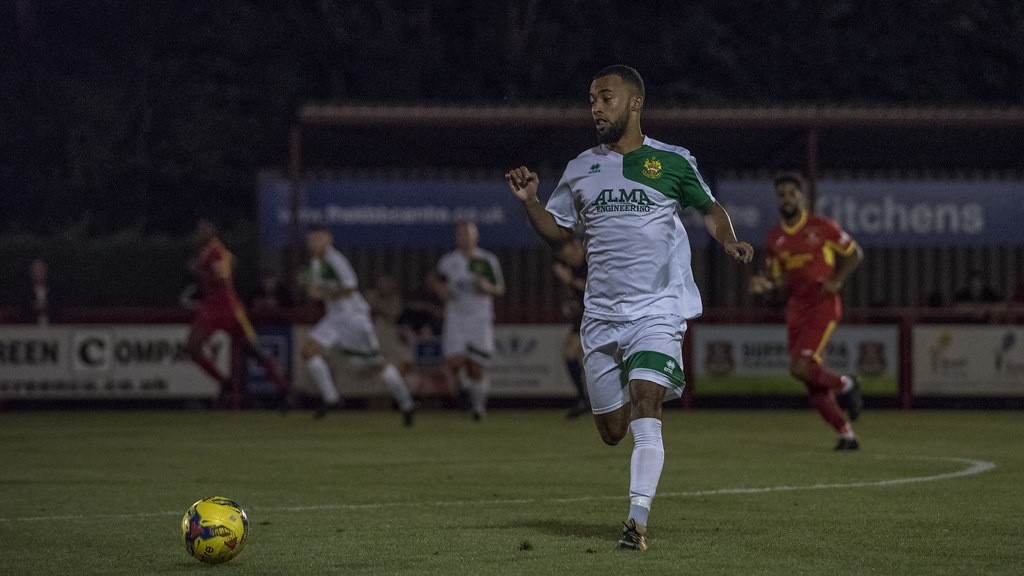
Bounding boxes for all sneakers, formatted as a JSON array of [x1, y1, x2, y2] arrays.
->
[[618, 518, 648, 551]]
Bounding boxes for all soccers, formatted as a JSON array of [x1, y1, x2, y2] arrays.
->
[[181, 495, 249, 565]]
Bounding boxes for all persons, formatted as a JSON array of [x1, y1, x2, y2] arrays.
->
[[423, 220, 507, 423], [302, 230, 417, 429], [503, 64, 755, 552], [19, 255, 55, 324], [182, 218, 286, 408], [746, 172, 863, 451], [955, 272, 996, 305]]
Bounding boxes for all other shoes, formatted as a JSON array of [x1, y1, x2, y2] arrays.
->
[[473, 411, 488, 421], [843, 375, 862, 420], [404, 408, 414, 427], [565, 396, 587, 420], [835, 438, 859, 452], [315, 397, 346, 418]]
